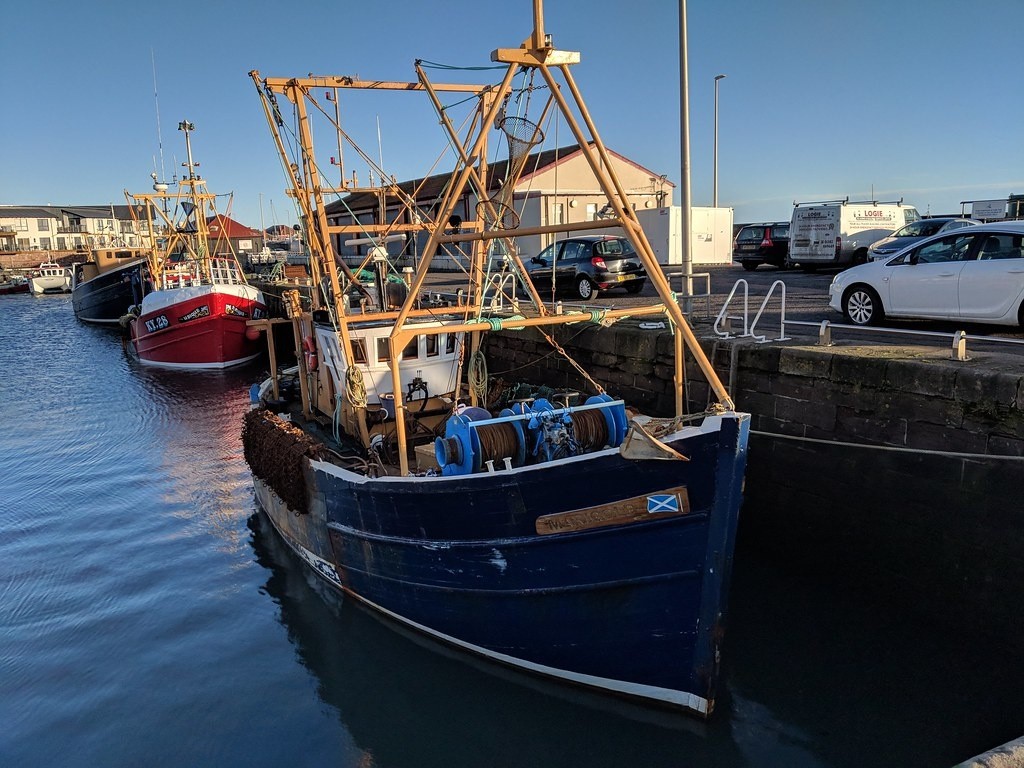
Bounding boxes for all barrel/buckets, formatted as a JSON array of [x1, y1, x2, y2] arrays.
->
[[378, 393, 407, 418]]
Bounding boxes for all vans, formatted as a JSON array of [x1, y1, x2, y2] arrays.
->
[[789, 197, 923, 266]]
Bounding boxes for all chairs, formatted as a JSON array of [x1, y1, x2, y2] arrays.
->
[[982, 238, 1007, 260], [565, 244, 580, 259], [1007, 237, 1022, 258]]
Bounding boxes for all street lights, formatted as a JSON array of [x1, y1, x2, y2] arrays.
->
[[714, 75, 727, 207]]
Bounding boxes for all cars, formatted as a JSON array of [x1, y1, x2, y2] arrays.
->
[[827, 220, 1024, 329], [513, 234, 646, 301], [867, 218, 983, 263]]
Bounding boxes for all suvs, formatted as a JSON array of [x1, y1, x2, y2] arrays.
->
[[733, 222, 796, 269]]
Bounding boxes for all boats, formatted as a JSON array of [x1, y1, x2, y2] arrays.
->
[[71, 247, 151, 326], [28, 251, 72, 297], [241, 1, 753, 721], [122, 118, 267, 370]]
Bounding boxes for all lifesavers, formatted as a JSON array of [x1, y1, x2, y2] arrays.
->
[[302, 336, 319, 372]]
